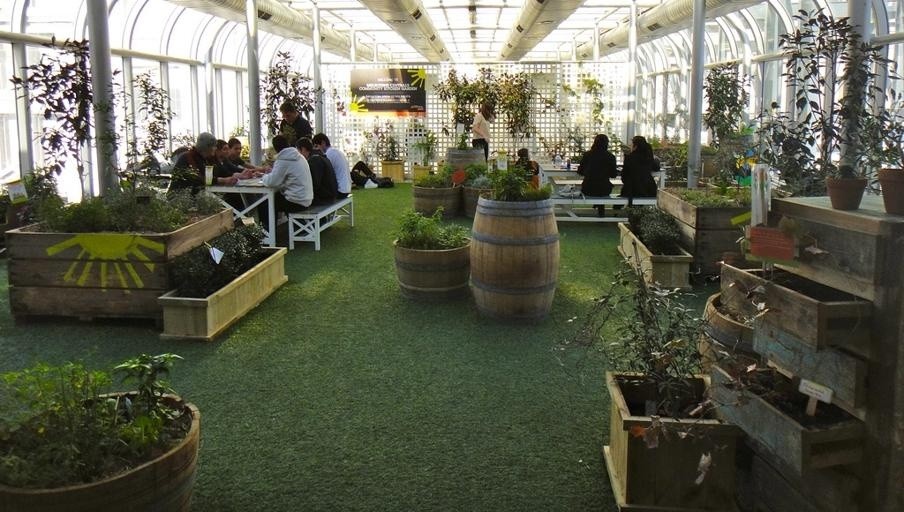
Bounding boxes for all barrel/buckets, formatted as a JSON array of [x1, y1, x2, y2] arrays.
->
[[0, 390, 201, 512], [460, 185, 496, 219], [470, 192, 561, 325], [392, 234, 472, 302], [445, 146, 486, 168], [411, 182, 462, 217], [720, 137, 752, 166]]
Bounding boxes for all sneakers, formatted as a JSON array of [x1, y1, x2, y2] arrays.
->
[[275, 211, 288, 227], [591, 208, 599, 217]]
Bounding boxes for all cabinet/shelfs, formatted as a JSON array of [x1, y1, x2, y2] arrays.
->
[[710, 193, 904, 511]]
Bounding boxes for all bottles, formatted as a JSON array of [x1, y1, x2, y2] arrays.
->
[[553, 154, 570, 168]]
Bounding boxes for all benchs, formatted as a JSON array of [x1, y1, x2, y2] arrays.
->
[[286, 193, 352, 250]]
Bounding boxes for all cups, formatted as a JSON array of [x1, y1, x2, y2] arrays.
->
[[204, 163, 213, 185]]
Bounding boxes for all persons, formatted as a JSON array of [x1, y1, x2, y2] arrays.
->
[[612, 136, 660, 210], [166, 102, 354, 232], [577, 134, 617, 215], [472, 102, 493, 161]]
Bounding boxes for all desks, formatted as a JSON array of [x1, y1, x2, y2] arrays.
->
[[539, 157, 667, 224], [203, 174, 281, 248]]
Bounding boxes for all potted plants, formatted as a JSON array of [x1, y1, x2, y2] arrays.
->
[[389, 206, 468, 299], [156, 222, 289, 342], [376, 131, 545, 222], [657, 61, 751, 278], [555, 253, 745, 512], [1, 170, 57, 247], [468, 161, 559, 317], [758, 6, 902, 218], [0, 350, 202, 512], [618, 204, 694, 292], [3, 184, 234, 329]]
